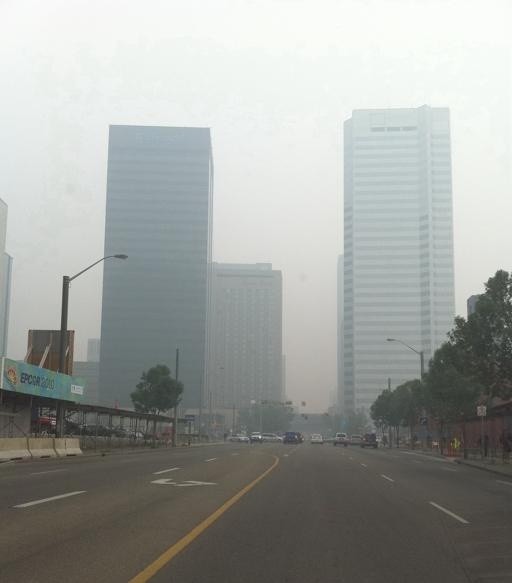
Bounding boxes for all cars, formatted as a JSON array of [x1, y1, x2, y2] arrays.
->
[[227, 431, 305, 444], [32, 412, 171, 446]]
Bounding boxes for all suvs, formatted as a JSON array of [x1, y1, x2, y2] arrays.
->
[[333, 432, 381, 448], [311, 433, 323, 444]]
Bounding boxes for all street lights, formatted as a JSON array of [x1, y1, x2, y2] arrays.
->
[[55, 253, 128, 438], [386, 338, 430, 452]]
